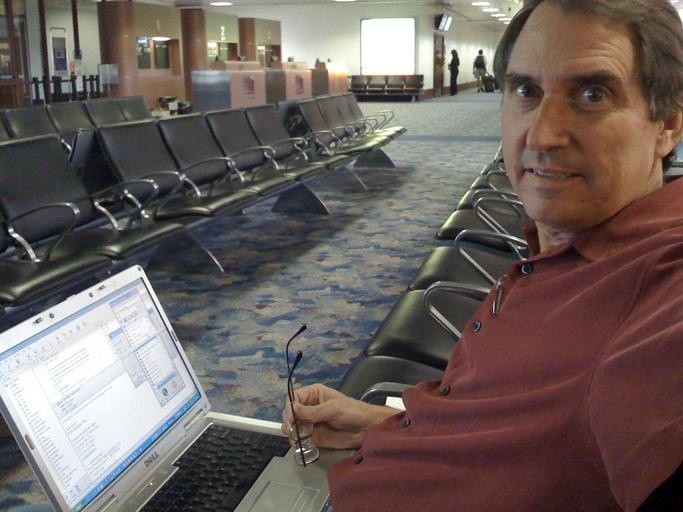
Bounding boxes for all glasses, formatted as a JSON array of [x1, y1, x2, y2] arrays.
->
[[284, 320, 319, 467]]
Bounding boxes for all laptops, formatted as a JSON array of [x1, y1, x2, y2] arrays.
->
[[0, 264, 359, 512]]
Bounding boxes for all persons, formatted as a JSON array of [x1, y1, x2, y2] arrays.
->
[[282, 0, 683, 512], [450, 50, 461, 95], [472, 49, 486, 92]]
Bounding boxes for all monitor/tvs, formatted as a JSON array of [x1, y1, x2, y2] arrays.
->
[[437, 14, 448, 30], [288, 57, 294, 62], [443, 16, 452, 31], [271, 57, 279, 61], [239, 56, 247, 61], [216, 56, 224, 61]]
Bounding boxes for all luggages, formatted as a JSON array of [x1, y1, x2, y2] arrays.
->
[[483, 70, 494, 92]]
